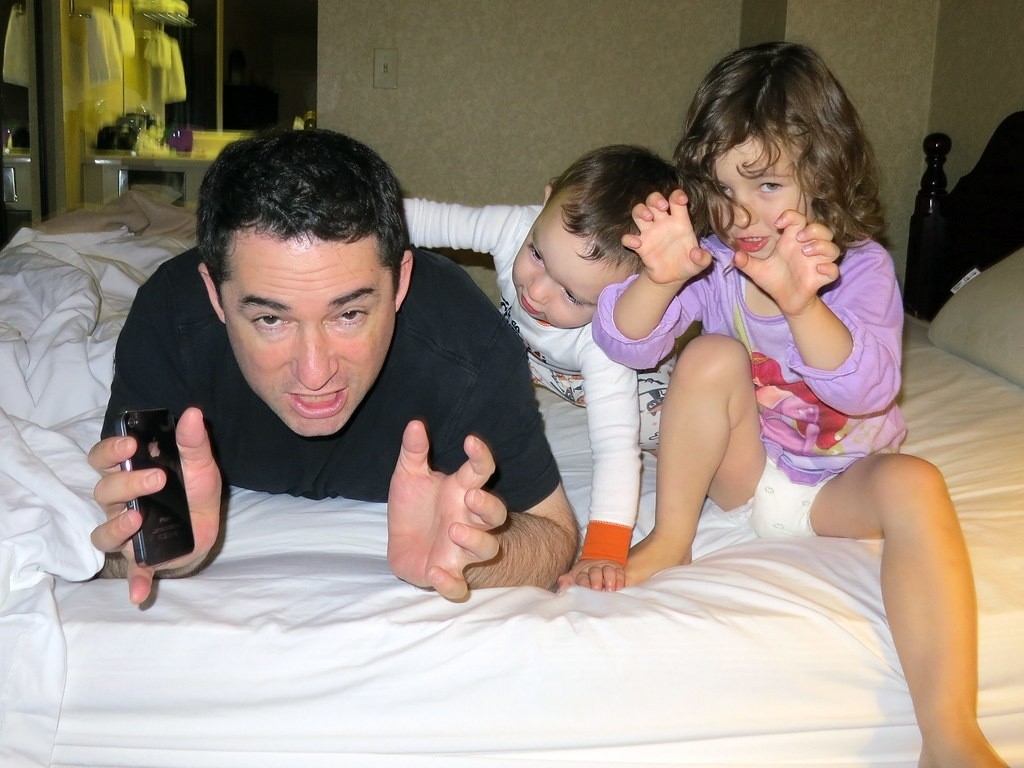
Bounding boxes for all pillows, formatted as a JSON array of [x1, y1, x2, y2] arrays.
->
[[927, 244, 1024, 389]]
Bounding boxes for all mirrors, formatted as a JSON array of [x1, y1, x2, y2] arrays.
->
[[0, 0, 42, 252], [123, 0, 318, 133]]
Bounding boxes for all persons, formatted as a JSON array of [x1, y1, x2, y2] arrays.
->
[[401, 145, 677, 594], [88, 125, 582, 605], [591, 42, 1006, 768]]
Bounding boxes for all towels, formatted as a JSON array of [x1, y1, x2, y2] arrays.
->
[[84, 7, 137, 85], [2, 4, 30, 88], [133, 0, 189, 18], [145, 29, 188, 104]]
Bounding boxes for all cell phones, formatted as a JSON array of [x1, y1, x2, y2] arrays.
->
[[116, 408, 195, 568]]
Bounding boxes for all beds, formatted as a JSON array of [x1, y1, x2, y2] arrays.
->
[[0, 112, 1024, 768]]
